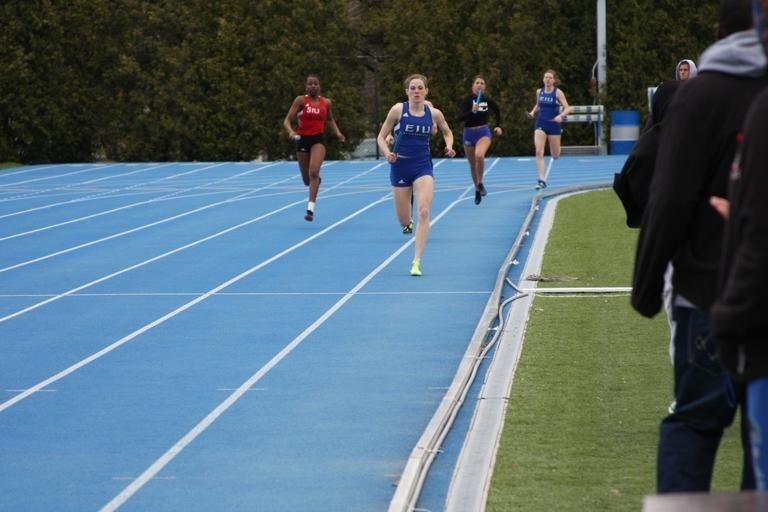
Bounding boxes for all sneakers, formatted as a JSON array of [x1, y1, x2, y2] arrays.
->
[[473, 190, 483, 206], [477, 182, 488, 197], [400, 218, 414, 235], [408, 258, 422, 276], [303, 208, 314, 222], [534, 179, 548, 191]]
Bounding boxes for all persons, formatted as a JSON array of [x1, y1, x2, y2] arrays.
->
[[385, 100, 438, 233], [527, 70, 570, 189], [283, 73, 346, 221], [457, 74, 503, 205], [376, 74, 456, 276]]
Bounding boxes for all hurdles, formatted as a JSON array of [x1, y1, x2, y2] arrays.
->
[[555, 105, 605, 155]]
